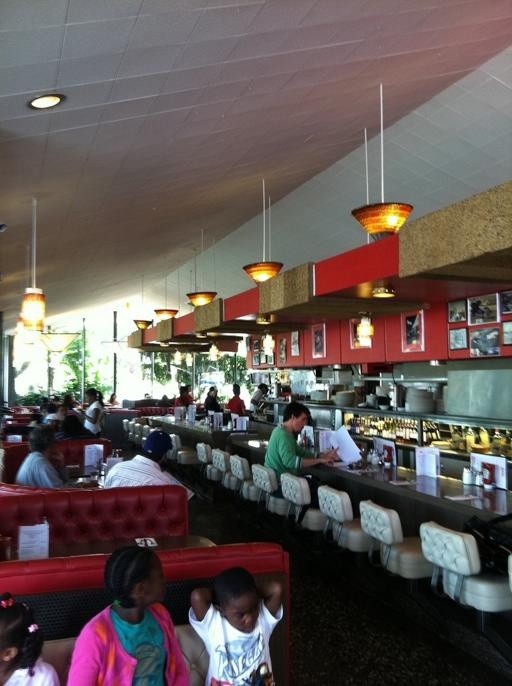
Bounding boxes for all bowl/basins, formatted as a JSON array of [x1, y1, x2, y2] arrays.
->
[[377, 404, 388, 410], [396, 406, 403, 412]]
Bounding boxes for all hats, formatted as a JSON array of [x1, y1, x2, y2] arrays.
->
[[141, 431, 172, 455]]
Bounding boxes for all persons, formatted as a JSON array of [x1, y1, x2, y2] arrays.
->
[[78, 388, 104, 439], [15, 424, 69, 490], [204, 387, 228, 426], [65, 546, 192, 686], [264, 402, 340, 524], [225, 384, 246, 416], [104, 431, 178, 489], [32, 392, 81, 416], [174, 384, 200, 407], [250, 383, 270, 412], [26, 414, 50, 431], [1, 591, 61, 686], [188, 567, 284, 686], [55, 415, 95, 440], [106, 393, 120, 406]]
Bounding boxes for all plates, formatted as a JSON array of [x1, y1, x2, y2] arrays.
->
[[404, 387, 435, 415], [334, 392, 353, 406]]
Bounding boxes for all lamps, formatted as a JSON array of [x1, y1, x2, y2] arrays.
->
[[134, 275, 153, 330], [186, 246, 219, 307], [18, 199, 46, 332], [154, 273, 179, 319], [159, 341, 170, 346], [208, 346, 219, 362], [242, 177, 284, 282], [351, 82, 414, 239], [373, 287, 397, 300]]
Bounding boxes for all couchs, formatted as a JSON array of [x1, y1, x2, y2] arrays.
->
[[0, 483, 194, 548], [0, 438, 116, 486], [0, 542, 286, 684]]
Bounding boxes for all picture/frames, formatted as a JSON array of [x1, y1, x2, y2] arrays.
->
[[277, 339, 287, 364], [261, 354, 266, 364], [290, 331, 300, 359], [401, 308, 428, 352], [468, 296, 498, 321], [252, 341, 259, 352], [466, 325, 498, 356], [351, 317, 374, 351], [498, 295, 512, 319], [253, 354, 259, 366], [310, 323, 328, 360], [444, 301, 466, 323], [501, 321, 512, 346], [449, 329, 463, 352]]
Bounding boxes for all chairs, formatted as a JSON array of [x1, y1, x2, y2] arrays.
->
[[251, 465, 304, 522], [197, 442, 225, 481], [213, 450, 253, 489], [121, 417, 162, 450], [280, 473, 336, 542], [232, 454, 274, 501], [317, 487, 382, 569], [165, 433, 199, 467], [357, 499, 433, 592], [420, 521, 510, 628]]
